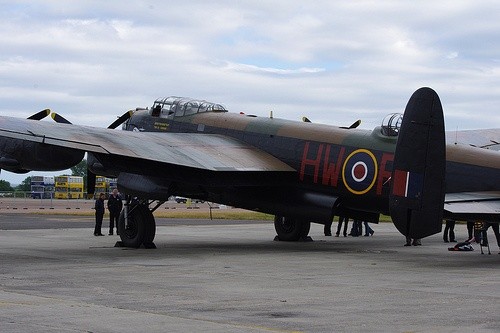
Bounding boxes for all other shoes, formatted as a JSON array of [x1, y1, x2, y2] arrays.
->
[[444, 240, 450, 242], [404, 244, 411, 246], [413, 243, 421, 245], [481, 243, 487, 246], [371, 231, 374, 236], [450, 240, 457, 242], [98, 234, 105, 236], [465, 239, 470, 242], [335, 233, 339, 237], [344, 235, 346, 237], [95, 233, 98, 236], [109, 233, 113, 235], [364, 235, 369, 237]]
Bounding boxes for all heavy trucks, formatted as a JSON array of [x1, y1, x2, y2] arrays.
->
[[30, 175, 55, 199], [93, 176, 108, 200], [54, 175, 84, 199], [106, 178, 126, 200]]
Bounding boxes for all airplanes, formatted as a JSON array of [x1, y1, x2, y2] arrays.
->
[[0, 85, 500, 247]]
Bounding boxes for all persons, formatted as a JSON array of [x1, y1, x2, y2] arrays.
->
[[324, 219, 332, 236], [107, 189, 122, 236], [465, 221, 500, 254], [364, 221, 374, 236], [335, 217, 349, 237], [348, 220, 362, 235], [404, 237, 422, 246], [443, 220, 457, 242], [94, 193, 105, 236]]
